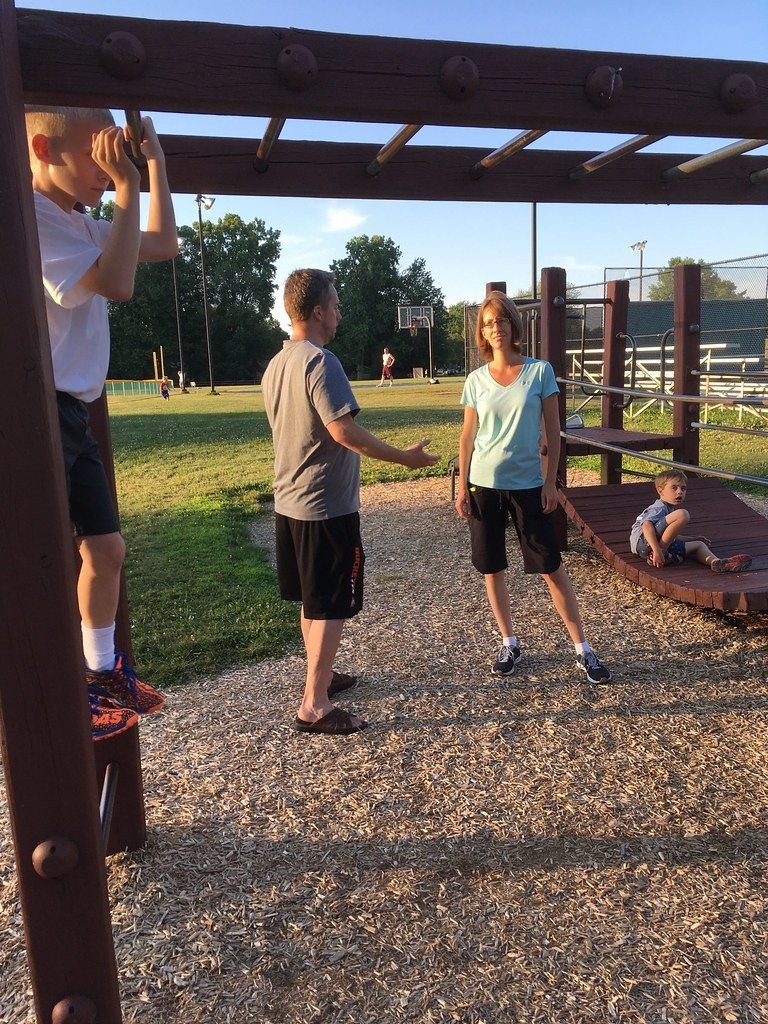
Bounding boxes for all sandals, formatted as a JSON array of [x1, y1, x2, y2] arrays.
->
[[711, 554, 753, 573], [647, 551, 684, 566]]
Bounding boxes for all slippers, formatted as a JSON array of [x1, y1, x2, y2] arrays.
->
[[300, 670, 356, 699], [293, 705, 368, 735]]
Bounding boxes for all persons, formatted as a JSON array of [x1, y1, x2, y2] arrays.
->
[[178, 370, 186, 389], [25, 105, 180, 742], [454, 290, 613, 684], [629, 470, 752, 572], [377, 348, 395, 387], [259, 269, 443, 736], [160, 376, 171, 401]]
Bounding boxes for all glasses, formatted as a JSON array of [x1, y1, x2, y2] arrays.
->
[[482, 317, 512, 328]]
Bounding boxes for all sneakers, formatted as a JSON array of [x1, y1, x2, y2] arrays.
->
[[87, 699, 139, 742], [490, 645, 521, 676], [84, 652, 166, 713], [577, 649, 611, 684]]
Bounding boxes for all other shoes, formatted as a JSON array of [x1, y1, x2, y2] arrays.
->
[[378, 384, 384, 387], [390, 383, 393, 387]]
